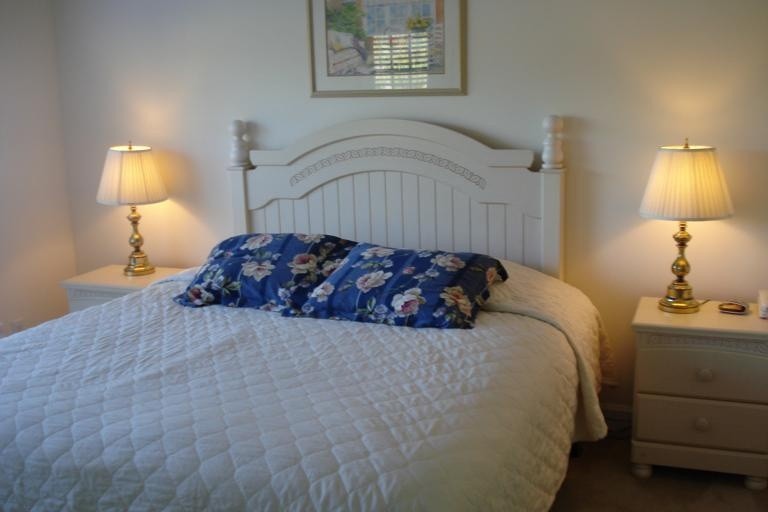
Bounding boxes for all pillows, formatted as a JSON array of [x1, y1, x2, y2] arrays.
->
[[297, 242, 508, 328], [172, 233, 358, 316]]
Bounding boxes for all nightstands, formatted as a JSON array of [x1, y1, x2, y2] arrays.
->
[[630, 296, 768, 492], [59, 265, 189, 313]]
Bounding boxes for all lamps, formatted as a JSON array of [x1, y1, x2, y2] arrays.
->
[[637, 136, 736, 313], [95, 141, 169, 276]]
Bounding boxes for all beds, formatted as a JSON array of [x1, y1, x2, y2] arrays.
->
[[0, 115, 618, 511]]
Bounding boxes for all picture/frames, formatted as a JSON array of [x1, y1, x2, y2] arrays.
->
[[306, 0, 468, 98]]
